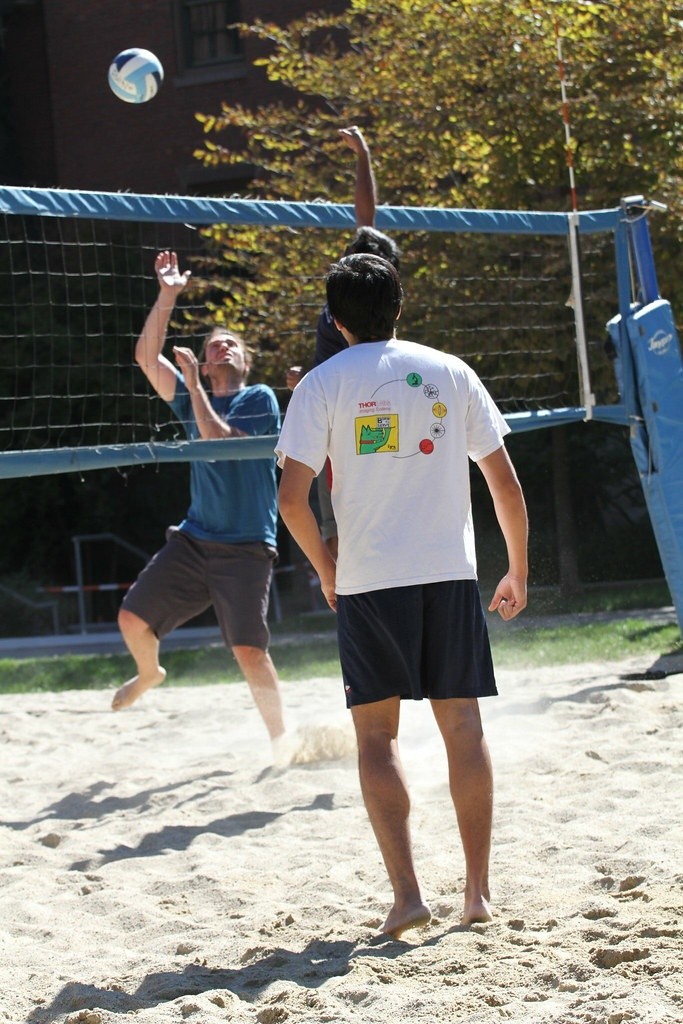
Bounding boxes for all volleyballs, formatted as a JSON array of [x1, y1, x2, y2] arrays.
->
[[109, 47, 166, 105]]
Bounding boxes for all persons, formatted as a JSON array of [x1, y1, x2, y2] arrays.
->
[[110, 250, 285, 739], [289, 124, 397, 565], [273, 253, 529, 940]]
